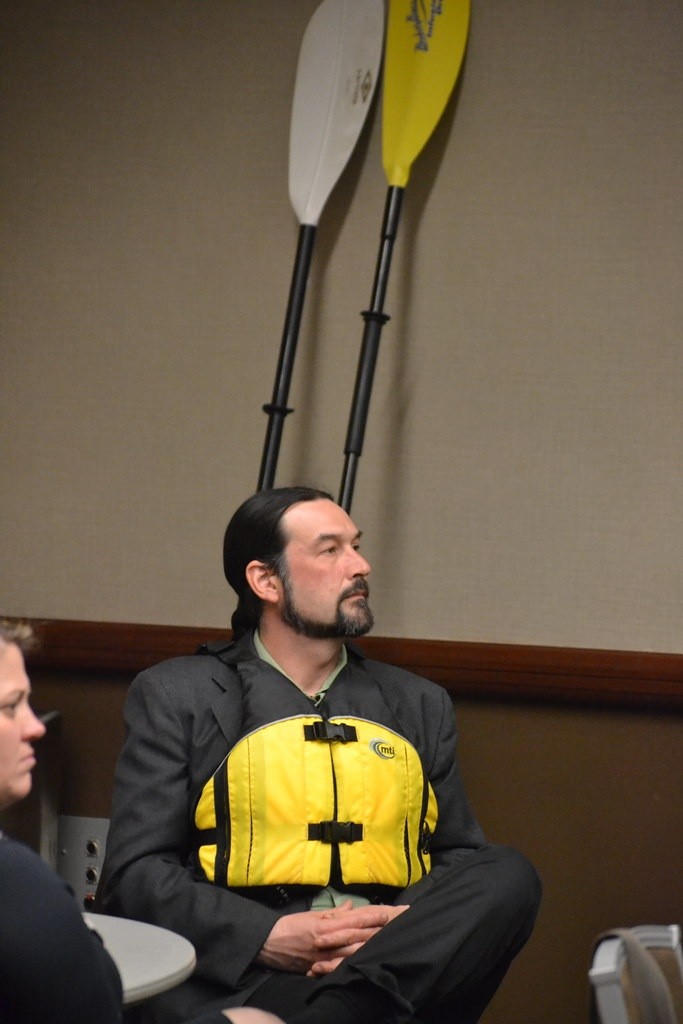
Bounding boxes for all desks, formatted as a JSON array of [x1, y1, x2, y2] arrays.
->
[[80, 911, 198, 1004]]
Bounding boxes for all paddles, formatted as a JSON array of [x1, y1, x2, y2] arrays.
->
[[258, 0, 385, 515], [337, 1, 473, 516]]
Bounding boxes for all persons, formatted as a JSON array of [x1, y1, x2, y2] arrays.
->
[[0, 618, 286, 1024], [100, 483, 543, 1024]]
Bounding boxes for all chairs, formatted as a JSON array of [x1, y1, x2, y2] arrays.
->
[[588, 925, 683, 1024]]
